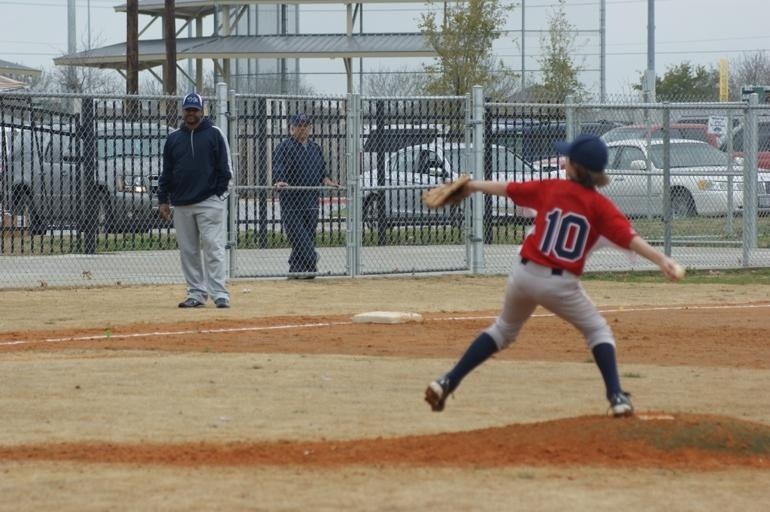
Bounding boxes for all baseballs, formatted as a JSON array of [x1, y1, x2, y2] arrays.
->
[[672, 264, 684, 279]]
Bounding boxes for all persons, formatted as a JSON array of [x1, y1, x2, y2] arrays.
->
[[271, 113, 339, 279], [421, 133, 687, 416], [156, 93, 234, 309]]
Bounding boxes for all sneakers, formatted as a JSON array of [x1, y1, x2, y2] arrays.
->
[[608, 394, 631, 416], [179, 298, 230, 308], [424, 379, 446, 411]]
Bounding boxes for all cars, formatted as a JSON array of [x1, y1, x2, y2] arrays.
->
[[0, 124, 174, 233]]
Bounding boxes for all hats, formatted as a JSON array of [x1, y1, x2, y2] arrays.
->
[[557, 135, 608, 170], [181, 92, 203, 110], [291, 114, 311, 126]]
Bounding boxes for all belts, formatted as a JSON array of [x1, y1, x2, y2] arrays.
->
[[520, 256, 562, 277]]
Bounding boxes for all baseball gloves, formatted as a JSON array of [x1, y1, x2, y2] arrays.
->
[[423, 175, 472, 210]]
